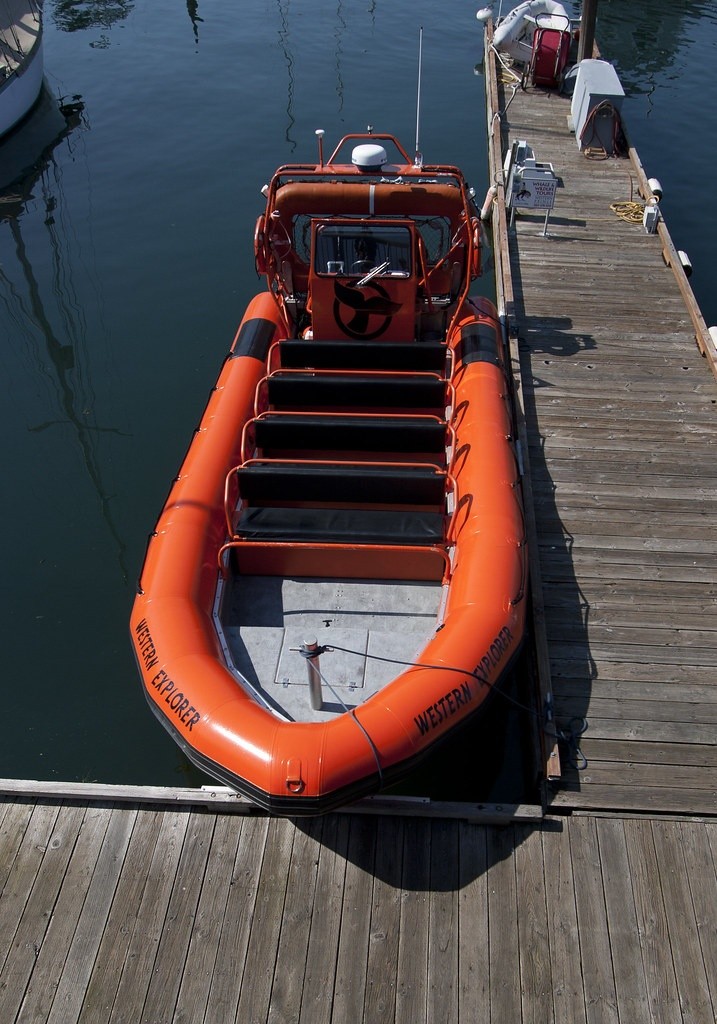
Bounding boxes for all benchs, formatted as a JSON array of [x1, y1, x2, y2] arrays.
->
[[224, 340, 458, 581]]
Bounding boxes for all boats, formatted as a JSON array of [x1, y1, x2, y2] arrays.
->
[[0, 0, 45, 136], [125, 128, 530, 816]]
